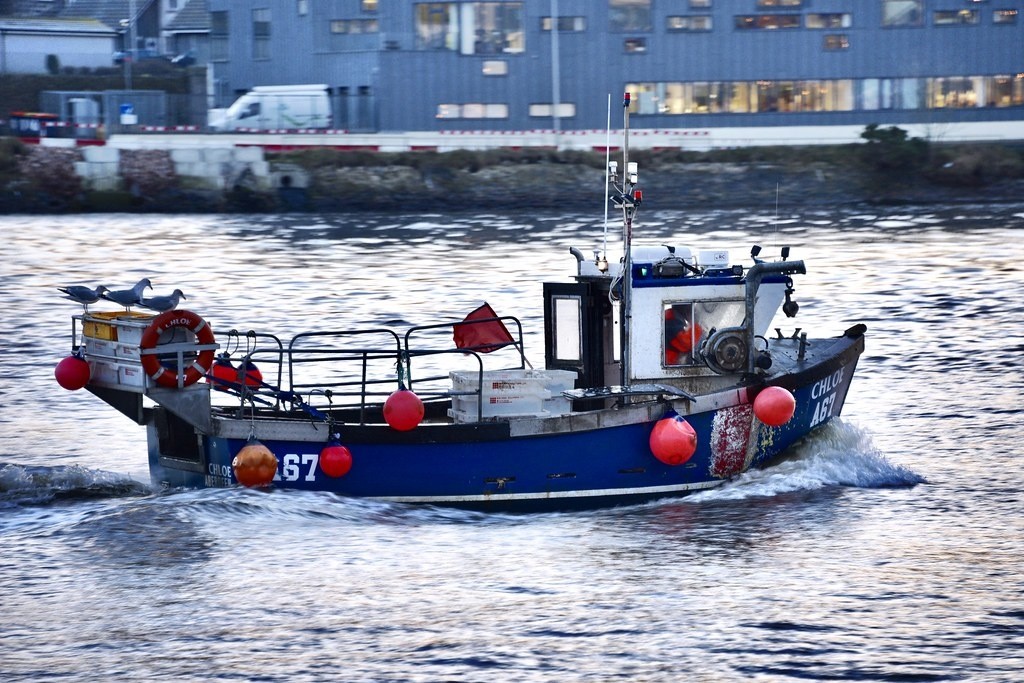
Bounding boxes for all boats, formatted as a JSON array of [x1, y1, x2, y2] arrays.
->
[[54, 92, 868, 513]]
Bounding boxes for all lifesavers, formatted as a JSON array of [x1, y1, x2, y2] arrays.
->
[[140, 310, 216, 388]]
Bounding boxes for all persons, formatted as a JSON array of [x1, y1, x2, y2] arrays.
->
[[665, 304, 701, 365]]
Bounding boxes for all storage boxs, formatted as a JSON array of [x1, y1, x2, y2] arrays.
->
[[448, 370, 579, 423], [79, 311, 197, 390]]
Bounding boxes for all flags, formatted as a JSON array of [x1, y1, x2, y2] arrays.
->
[[453, 303, 512, 357]]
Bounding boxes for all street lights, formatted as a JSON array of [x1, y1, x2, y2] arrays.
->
[[120, 17, 137, 62]]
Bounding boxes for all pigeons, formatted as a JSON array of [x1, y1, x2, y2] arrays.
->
[[57, 278, 187, 315]]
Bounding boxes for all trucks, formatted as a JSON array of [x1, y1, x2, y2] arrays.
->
[[211, 84, 333, 130]]
